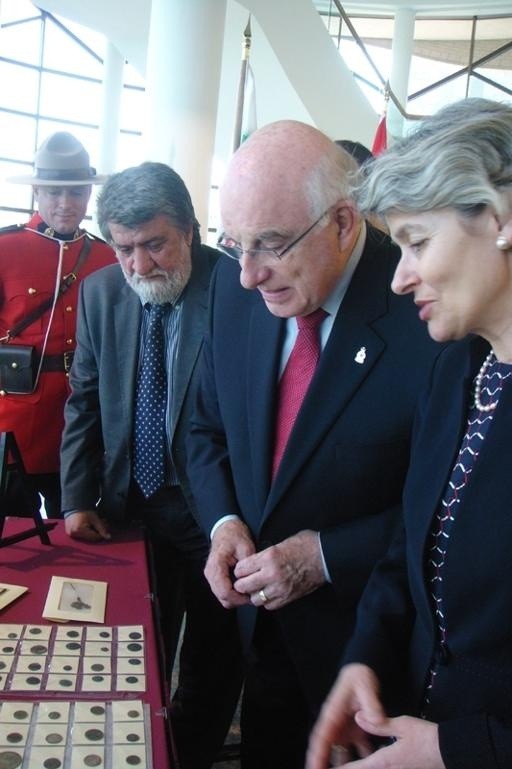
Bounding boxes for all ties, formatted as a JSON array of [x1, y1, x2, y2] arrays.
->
[[131, 304, 167, 500], [271, 307, 330, 482]]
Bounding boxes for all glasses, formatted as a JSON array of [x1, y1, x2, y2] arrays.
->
[[217, 211, 328, 266]]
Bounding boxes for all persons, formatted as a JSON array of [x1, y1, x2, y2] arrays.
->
[[179, 119, 451, 769], [303, 96, 511, 769], [332, 140, 390, 239], [61, 162, 245, 769], [1, 132, 121, 520]]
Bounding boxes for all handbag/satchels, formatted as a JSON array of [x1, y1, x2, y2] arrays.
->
[[0, 344, 35, 396]]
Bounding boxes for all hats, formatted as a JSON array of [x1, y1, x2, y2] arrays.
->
[[6, 131, 109, 186]]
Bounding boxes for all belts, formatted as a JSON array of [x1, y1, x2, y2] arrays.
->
[[35, 351, 74, 372]]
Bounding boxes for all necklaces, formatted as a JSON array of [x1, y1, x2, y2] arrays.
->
[[474, 348, 508, 412]]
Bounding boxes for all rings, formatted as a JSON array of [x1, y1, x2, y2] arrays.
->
[[331, 744, 349, 754], [259, 589, 268, 603]]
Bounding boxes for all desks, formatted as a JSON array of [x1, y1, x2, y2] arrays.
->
[[1, 512, 170, 768]]
